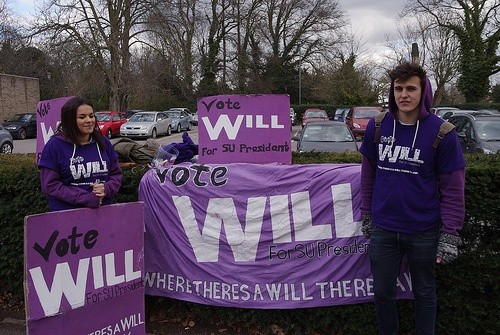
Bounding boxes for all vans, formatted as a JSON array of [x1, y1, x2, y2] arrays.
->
[[169, 107, 194, 126], [93, 111, 128, 139]]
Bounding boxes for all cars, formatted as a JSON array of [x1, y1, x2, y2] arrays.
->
[[0, 124, 14, 153], [290, 108, 297, 126], [163, 110, 193, 133], [346, 106, 382, 140], [119, 112, 172, 139], [127, 110, 143, 119], [192, 110, 198, 126], [441, 110, 500, 153], [338, 108, 351, 122], [302, 110, 330, 129], [292, 121, 359, 154], [333, 107, 346, 121], [1, 113, 37, 140], [430, 107, 460, 120], [301, 108, 320, 117]]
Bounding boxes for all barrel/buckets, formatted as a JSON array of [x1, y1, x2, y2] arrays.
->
[[151, 143, 178, 169]]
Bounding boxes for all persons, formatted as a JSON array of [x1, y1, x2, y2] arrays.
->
[[359, 61, 466, 335], [38, 96, 123, 212]]
